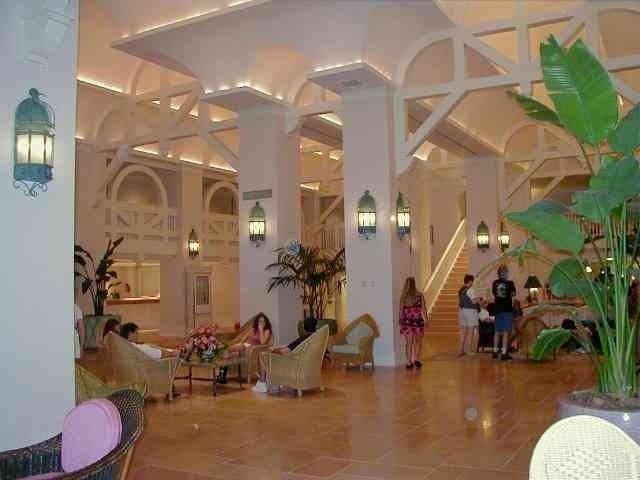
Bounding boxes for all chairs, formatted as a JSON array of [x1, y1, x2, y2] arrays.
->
[[477, 308, 504, 354], [521, 316, 559, 361], [529, 413, 640, 480], [102, 312, 380, 400], [0, 388, 145, 480]]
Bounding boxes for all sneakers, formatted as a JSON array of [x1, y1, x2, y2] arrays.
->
[[501, 353, 512, 360], [415, 361, 421, 367], [407, 362, 413, 368], [492, 352, 498, 358], [251, 380, 268, 392]]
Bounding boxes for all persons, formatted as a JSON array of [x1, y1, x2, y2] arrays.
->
[[218, 311, 272, 383], [398, 276, 428, 369], [102, 318, 121, 339], [577, 326, 596, 354], [476, 296, 495, 323], [250, 316, 318, 393], [455, 273, 488, 357], [491, 262, 517, 361], [74, 303, 85, 359], [119, 322, 181, 397]]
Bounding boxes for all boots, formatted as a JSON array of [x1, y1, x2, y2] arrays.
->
[[216, 367, 227, 384]]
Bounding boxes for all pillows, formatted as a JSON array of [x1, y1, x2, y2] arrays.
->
[[60, 398, 122, 473]]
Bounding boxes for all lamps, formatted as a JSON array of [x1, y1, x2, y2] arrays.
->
[[357, 191, 377, 239], [500, 226, 510, 248], [396, 192, 411, 239], [477, 220, 490, 248], [248, 200, 265, 245], [523, 276, 543, 303], [189, 229, 200, 259], [13, 88, 56, 198]]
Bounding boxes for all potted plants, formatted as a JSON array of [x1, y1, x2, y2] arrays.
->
[[504, 34, 640, 451], [264, 239, 346, 362], [75, 236, 122, 351]]
